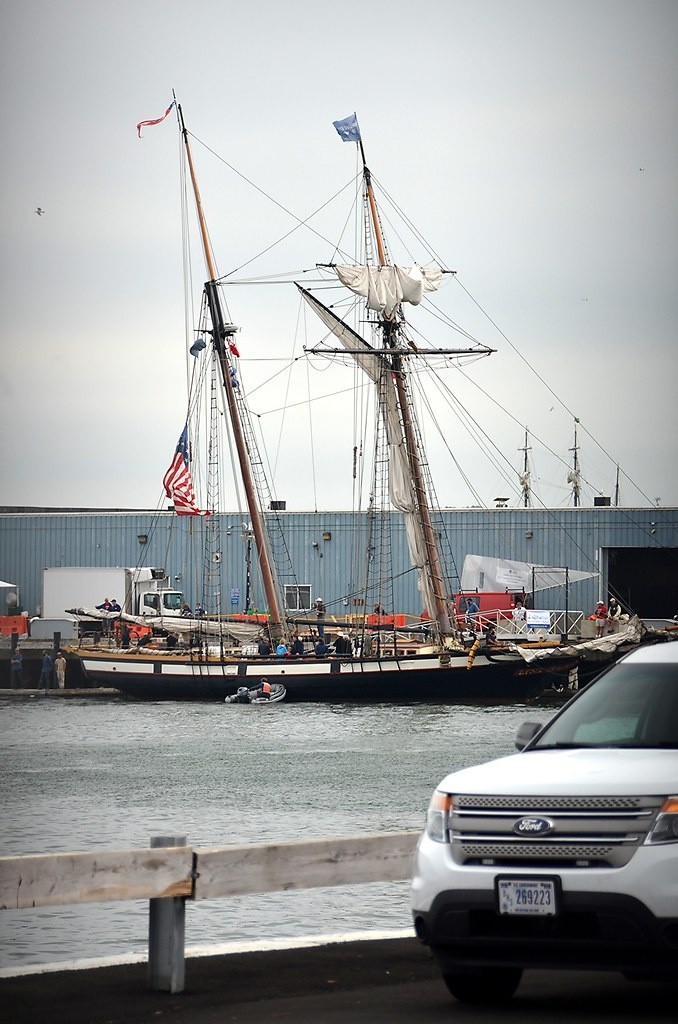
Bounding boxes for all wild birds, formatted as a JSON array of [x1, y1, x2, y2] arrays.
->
[[34, 207, 45, 216]]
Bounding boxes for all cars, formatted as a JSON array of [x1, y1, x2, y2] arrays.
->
[[411, 636, 678, 1005]]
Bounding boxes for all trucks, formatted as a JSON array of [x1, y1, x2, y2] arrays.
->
[[43, 567, 193, 632]]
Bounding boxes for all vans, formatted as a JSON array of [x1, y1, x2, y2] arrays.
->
[[421, 592, 533, 632]]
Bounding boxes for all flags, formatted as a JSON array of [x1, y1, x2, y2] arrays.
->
[[227, 338, 240, 357], [137, 100, 174, 139], [190, 338, 206, 357], [332, 113, 362, 142], [222, 368, 239, 387], [164, 425, 210, 516]]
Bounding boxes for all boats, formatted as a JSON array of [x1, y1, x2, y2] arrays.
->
[[225, 683, 286, 705]]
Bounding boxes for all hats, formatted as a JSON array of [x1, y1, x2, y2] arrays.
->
[[316, 598, 322, 601], [610, 598, 615, 601], [110, 599, 116, 602], [597, 601, 603, 604], [337, 631, 343, 637], [293, 634, 298, 637]]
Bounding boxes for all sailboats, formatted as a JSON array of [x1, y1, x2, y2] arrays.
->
[[60, 104, 678, 702]]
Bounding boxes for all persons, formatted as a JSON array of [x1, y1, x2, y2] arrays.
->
[[605, 598, 621, 633], [511, 602, 527, 634], [11, 647, 24, 690], [594, 601, 608, 638], [166, 632, 179, 650], [485, 625, 500, 646], [180, 604, 192, 617], [122, 628, 133, 649], [248, 678, 271, 699], [373, 602, 388, 616], [467, 598, 480, 633], [54, 652, 67, 689], [195, 602, 204, 619], [110, 599, 122, 629], [260, 631, 372, 659], [137, 632, 153, 647], [36, 650, 54, 689], [315, 598, 326, 636], [95, 598, 112, 638]]
